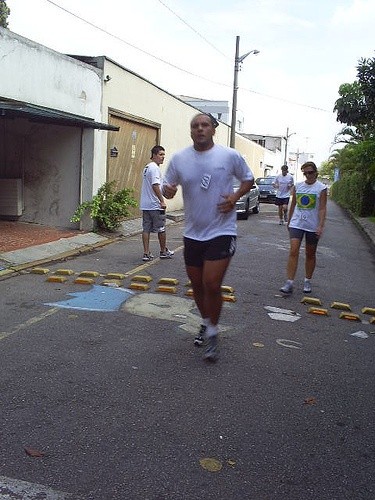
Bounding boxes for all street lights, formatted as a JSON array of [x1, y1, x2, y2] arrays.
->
[[284, 128, 296, 166], [230, 36, 261, 149]]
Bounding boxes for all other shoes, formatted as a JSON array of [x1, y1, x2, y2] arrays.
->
[[303, 280, 312, 293], [278, 220, 284, 225], [280, 281, 293, 293], [284, 214, 288, 222], [142, 251, 154, 260], [159, 247, 174, 258]]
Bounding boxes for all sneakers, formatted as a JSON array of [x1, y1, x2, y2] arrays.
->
[[203, 333, 219, 361], [194, 324, 207, 346]]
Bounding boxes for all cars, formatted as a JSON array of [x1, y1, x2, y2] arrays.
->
[[232, 176, 261, 220], [255, 176, 279, 203]]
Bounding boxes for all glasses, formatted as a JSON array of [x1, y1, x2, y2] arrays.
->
[[303, 170, 315, 175]]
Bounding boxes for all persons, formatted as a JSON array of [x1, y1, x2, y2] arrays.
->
[[279, 162, 327, 294], [272, 165, 294, 225], [140, 145, 175, 261], [160, 114, 255, 361]]
